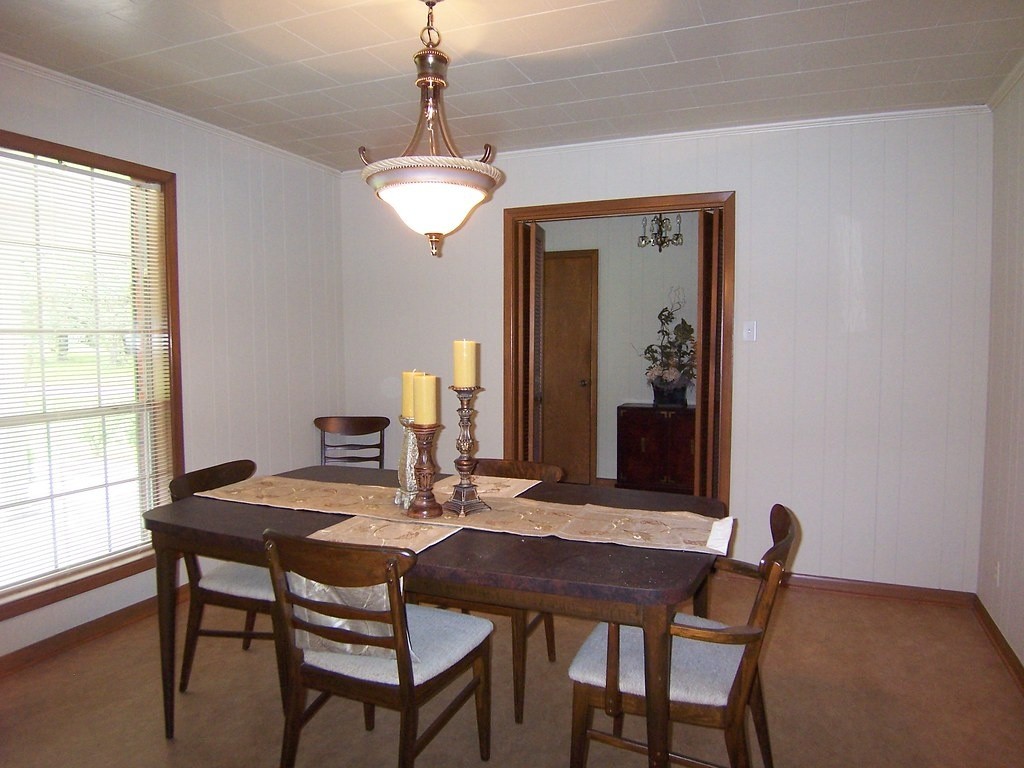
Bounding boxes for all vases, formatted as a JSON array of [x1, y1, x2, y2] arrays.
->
[[648, 380, 688, 408]]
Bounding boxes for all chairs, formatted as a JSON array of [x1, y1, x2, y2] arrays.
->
[[313, 415, 391, 469], [260, 527, 494, 768], [168, 459, 276, 693], [568, 502, 797, 768], [403, 456, 563, 724]]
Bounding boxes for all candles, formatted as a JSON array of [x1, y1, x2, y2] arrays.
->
[[452, 339, 477, 387], [413, 372, 437, 425], [401, 368, 422, 419]]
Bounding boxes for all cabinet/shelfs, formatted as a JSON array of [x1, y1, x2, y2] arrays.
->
[[613, 403, 697, 496]]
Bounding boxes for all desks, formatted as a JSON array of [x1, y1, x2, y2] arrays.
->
[[138, 461, 728, 768]]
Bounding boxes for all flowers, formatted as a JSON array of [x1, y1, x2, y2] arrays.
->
[[635, 286, 698, 387]]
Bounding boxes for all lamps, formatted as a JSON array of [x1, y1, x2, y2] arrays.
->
[[358, 0, 503, 256], [637, 212, 685, 253]]
